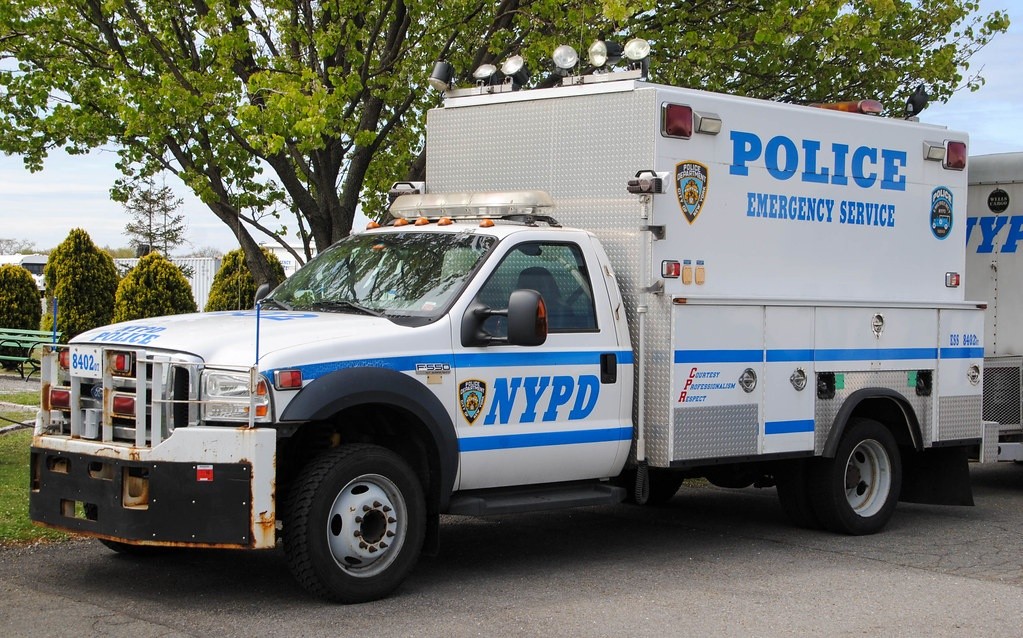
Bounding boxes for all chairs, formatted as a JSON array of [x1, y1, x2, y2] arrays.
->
[[0, 328, 64, 382], [515, 267, 579, 329]]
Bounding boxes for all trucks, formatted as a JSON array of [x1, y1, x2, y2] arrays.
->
[[28, 34, 998, 605], [960, 151, 1023, 467]]
